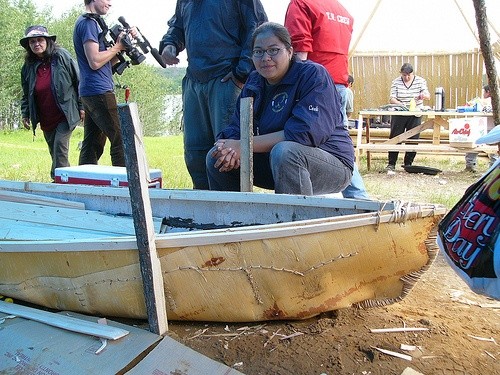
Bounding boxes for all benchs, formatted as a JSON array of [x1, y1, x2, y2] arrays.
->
[[357, 144, 495, 173]]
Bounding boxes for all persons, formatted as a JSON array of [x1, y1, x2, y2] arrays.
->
[[19, 25, 86, 183], [346, 75, 355, 120], [283, 0, 368, 199], [379, 62, 430, 173], [479, 85, 493, 112], [71, 0, 139, 167], [205, 22, 357, 196], [158, 0, 268, 192]]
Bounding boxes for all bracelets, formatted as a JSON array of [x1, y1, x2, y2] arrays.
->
[[82, 110, 85, 113]]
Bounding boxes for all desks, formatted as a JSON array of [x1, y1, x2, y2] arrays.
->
[[357, 110, 493, 173]]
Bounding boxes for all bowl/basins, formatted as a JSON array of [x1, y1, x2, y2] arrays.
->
[[445, 109, 456, 113]]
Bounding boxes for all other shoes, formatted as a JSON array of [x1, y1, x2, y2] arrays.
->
[[385, 164, 395, 170]]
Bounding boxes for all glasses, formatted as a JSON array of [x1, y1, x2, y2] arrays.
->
[[252, 47, 288, 56]]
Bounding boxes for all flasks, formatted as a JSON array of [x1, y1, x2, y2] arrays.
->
[[435, 87, 444, 111]]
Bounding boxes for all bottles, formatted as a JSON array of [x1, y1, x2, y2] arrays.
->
[[409, 97, 416, 112]]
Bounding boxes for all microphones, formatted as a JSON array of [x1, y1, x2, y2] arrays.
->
[[136, 26, 167, 69]]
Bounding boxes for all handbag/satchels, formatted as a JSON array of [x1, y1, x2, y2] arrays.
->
[[436, 123, 499, 301]]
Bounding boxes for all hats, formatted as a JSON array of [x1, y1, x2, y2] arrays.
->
[[20, 24, 56, 50]]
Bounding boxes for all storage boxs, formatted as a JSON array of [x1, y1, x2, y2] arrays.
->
[[448, 117, 493, 142], [55, 164, 162, 188]]
